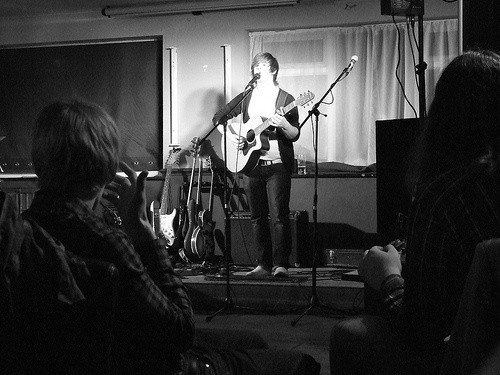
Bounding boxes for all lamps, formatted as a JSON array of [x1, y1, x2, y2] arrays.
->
[[101, 0, 300, 18]]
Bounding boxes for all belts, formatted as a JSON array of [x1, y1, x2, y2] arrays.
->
[[259, 158, 282, 166]]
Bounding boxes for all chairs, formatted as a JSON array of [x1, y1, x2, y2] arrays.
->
[[0, 255, 119, 375], [332, 239, 500, 375]]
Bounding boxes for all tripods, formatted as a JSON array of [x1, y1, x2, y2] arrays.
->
[[290, 68, 347, 325], [197, 89, 259, 320]]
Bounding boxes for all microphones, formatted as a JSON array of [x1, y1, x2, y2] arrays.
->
[[345, 55, 358, 74], [245, 73, 260, 90]]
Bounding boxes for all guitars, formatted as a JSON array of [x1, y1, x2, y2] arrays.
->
[[150, 145, 177, 246], [181, 157, 196, 236], [183, 156, 203, 255], [220, 87, 316, 173], [190, 156, 216, 258]]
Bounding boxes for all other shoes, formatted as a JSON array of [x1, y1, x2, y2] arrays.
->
[[246, 266, 270, 276], [274, 266, 288, 277]]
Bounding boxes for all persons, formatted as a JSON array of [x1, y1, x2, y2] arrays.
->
[[330, 50, 500, 375], [212, 52, 300, 278], [23, 100, 321, 375]]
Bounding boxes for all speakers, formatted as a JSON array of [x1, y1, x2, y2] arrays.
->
[[375, 118, 422, 244], [381, 0, 424, 16], [223, 210, 309, 268]]
